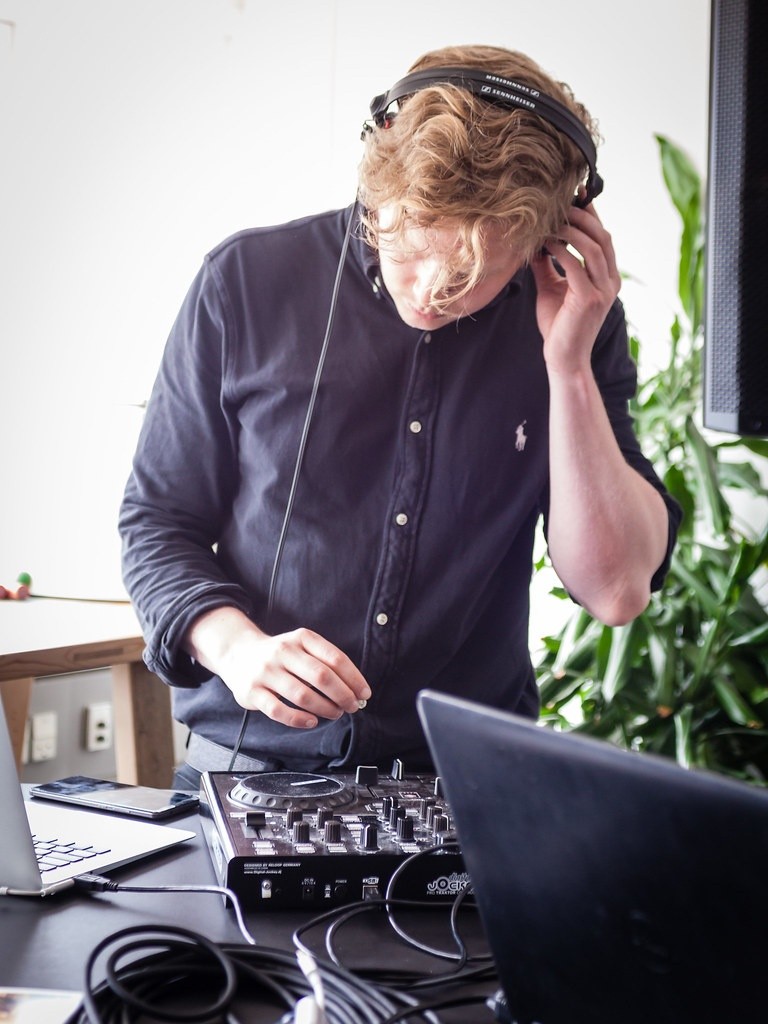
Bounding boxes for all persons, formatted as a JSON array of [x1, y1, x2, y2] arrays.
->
[[117, 45, 681, 791]]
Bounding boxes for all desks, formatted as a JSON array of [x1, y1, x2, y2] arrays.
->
[[0, 593, 176, 789]]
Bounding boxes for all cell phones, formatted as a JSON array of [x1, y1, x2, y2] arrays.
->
[[30, 776, 200, 820]]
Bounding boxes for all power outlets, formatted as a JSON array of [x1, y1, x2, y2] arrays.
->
[[21, 703, 111, 765]]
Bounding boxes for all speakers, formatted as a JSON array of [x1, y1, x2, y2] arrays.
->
[[701, 0, 768, 435]]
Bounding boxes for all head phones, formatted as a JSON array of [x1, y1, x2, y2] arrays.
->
[[360, 68, 604, 257]]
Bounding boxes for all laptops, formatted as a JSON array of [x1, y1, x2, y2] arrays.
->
[[418, 690, 768, 1024], [0, 699, 197, 895]]
